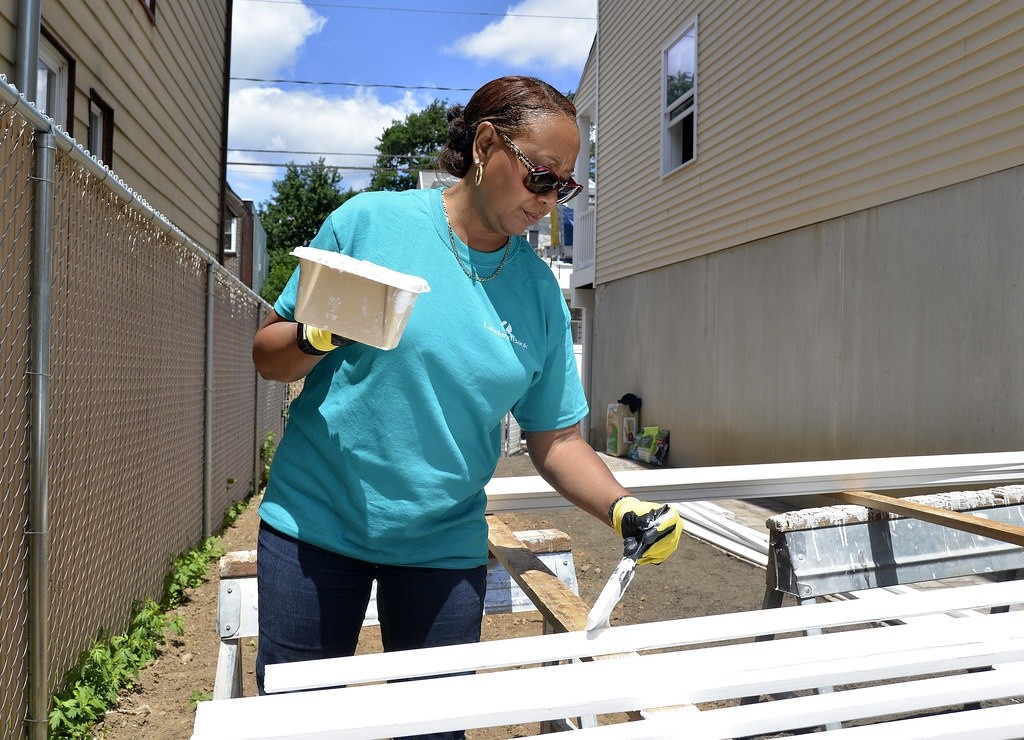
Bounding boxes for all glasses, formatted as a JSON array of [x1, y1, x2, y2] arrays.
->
[[494, 128, 583, 205]]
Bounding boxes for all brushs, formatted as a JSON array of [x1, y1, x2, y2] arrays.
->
[[584, 503, 671, 632]]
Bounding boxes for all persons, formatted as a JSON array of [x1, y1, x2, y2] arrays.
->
[[253, 75, 683, 740]]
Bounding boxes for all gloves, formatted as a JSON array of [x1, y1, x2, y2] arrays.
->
[[297, 323, 357, 356], [613, 496, 683, 566]]
